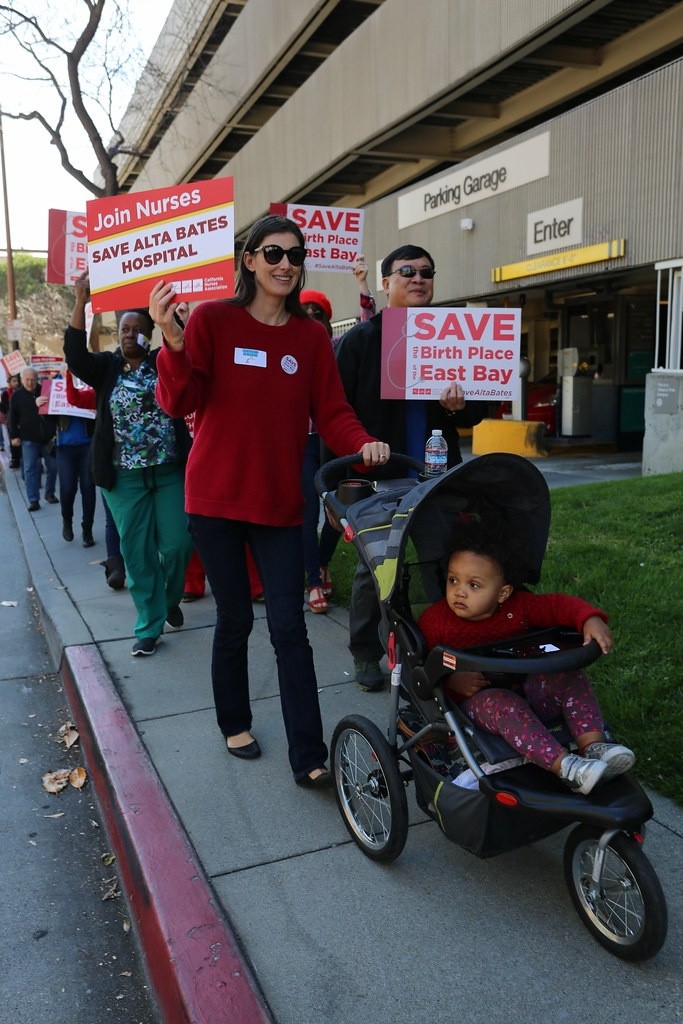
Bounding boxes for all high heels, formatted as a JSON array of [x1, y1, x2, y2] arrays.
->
[[319, 565, 333, 597], [308, 586, 328, 613]]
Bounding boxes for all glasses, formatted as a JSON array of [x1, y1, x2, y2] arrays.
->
[[387, 264, 436, 279], [252, 244, 308, 266]]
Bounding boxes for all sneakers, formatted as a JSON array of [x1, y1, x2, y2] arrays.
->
[[583, 741, 636, 775], [132, 634, 162, 657], [557, 753, 607, 794], [353, 656, 386, 692], [163, 604, 184, 629]]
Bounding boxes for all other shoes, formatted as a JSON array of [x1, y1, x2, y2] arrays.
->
[[9, 458, 21, 468], [295, 764, 335, 789], [257, 591, 265, 604], [225, 732, 261, 759], [27, 500, 40, 512], [181, 591, 203, 602], [44, 493, 59, 504]]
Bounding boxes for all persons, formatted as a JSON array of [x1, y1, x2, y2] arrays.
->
[[148, 216, 390, 792], [0, 255, 377, 657], [333, 246, 486, 691], [417, 537, 635, 794]]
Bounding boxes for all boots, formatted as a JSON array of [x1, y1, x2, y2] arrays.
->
[[100, 555, 126, 591], [81, 522, 95, 548], [62, 517, 74, 542]]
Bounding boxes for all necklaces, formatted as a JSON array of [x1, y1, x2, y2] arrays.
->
[[275, 312, 289, 326]]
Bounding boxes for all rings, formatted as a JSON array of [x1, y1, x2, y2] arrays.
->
[[378, 455, 386, 458]]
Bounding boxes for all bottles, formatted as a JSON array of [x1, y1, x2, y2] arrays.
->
[[425, 430, 448, 476]]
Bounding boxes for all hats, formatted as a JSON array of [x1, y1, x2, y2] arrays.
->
[[298, 289, 334, 318]]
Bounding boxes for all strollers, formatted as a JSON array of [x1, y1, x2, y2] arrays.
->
[[312, 448, 669, 964]]
